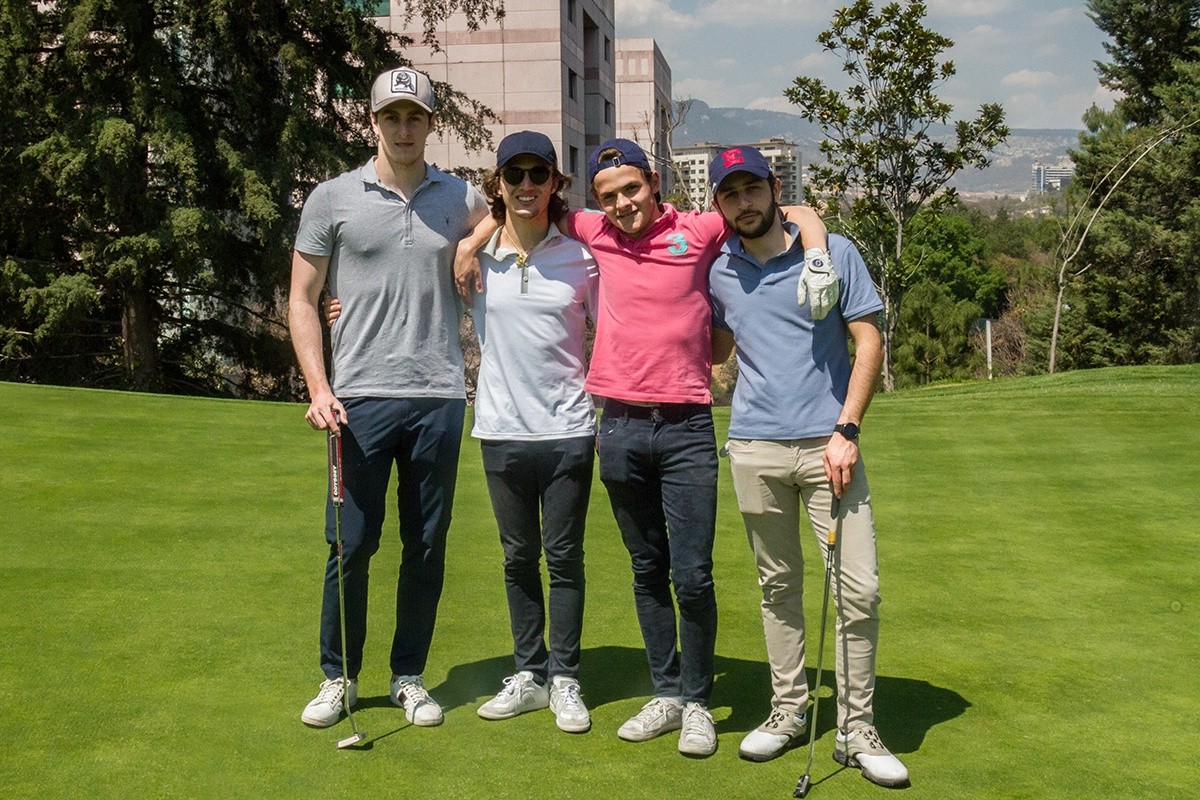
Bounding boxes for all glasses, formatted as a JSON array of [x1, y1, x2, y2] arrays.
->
[[498, 166, 556, 186]]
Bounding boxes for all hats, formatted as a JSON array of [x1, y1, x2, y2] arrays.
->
[[708, 147, 770, 194], [371, 66, 434, 112], [497, 131, 558, 165], [589, 140, 649, 183]]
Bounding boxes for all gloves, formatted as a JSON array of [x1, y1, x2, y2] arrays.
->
[[798, 248, 841, 320]]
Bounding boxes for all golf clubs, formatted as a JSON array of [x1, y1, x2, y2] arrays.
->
[[326, 407, 368, 750], [790, 478, 842, 800]]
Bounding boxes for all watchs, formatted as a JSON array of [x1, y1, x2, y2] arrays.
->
[[834, 423, 860, 439]]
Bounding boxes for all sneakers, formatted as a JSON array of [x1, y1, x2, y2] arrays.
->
[[679, 703, 717, 754], [301, 677, 358, 726], [549, 678, 591, 732], [389, 675, 443, 725], [617, 697, 682, 742], [477, 671, 549, 720], [739, 711, 807, 762], [833, 726, 909, 787]]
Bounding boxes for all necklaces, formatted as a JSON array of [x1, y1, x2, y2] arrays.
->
[[503, 226, 543, 265]]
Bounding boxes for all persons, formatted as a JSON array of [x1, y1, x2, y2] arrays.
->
[[289, 66, 489, 726], [455, 140, 838, 756], [321, 129, 599, 733], [704, 145, 910, 788]]
